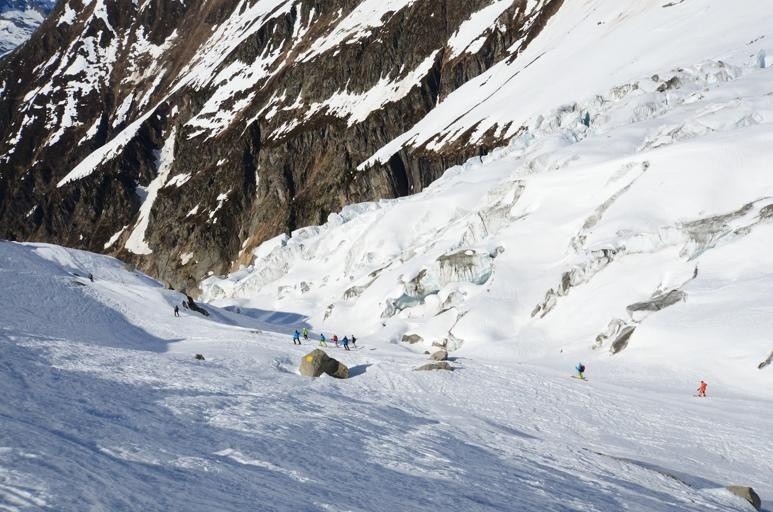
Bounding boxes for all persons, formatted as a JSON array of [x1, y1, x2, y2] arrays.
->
[[696, 380, 708, 397], [293, 327, 357, 350], [174, 305, 179, 316], [575, 362, 584, 379], [88, 273, 94, 282]]
